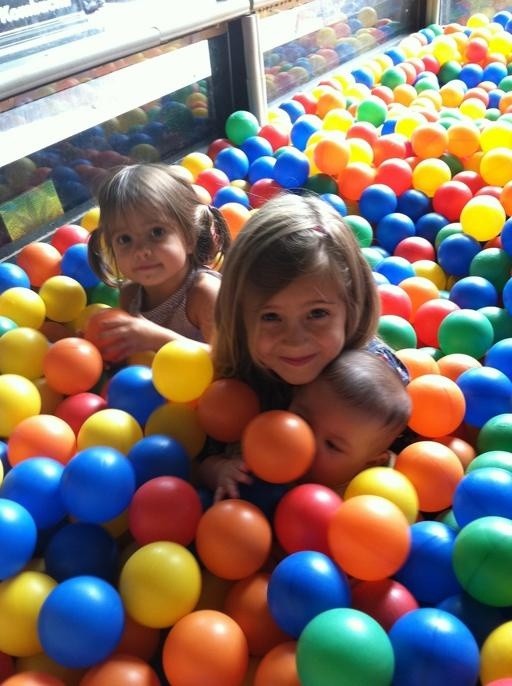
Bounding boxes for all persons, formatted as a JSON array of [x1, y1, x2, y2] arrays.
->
[[195, 193, 405, 502], [87, 164, 231, 363], [287, 347, 411, 483]]
